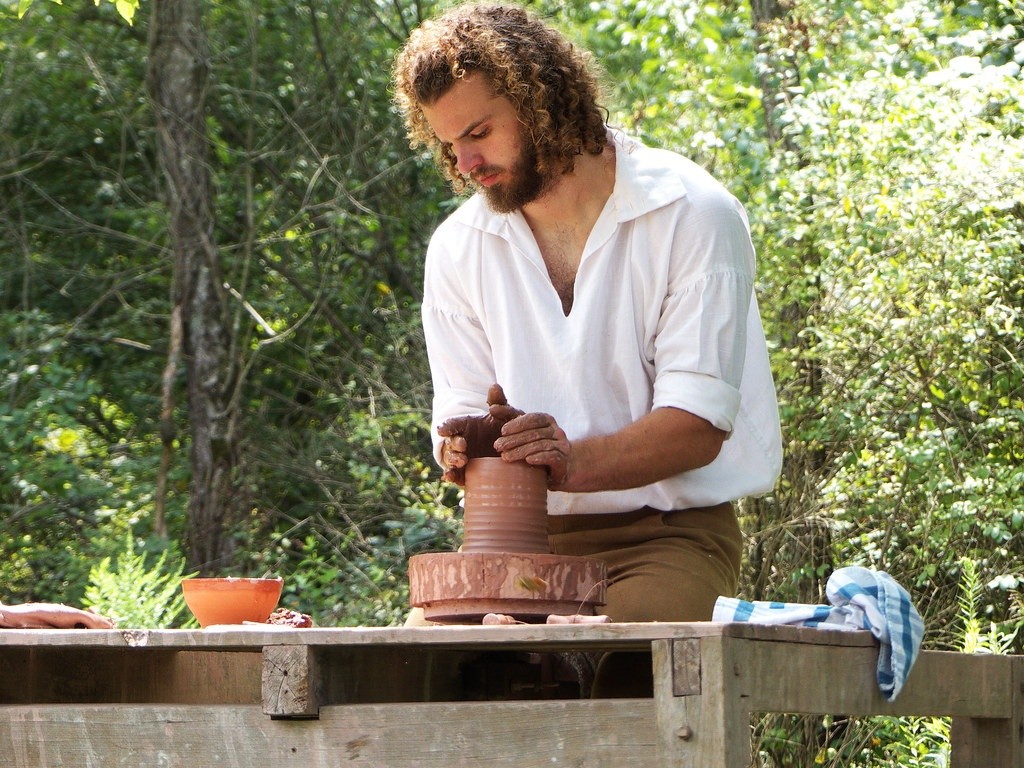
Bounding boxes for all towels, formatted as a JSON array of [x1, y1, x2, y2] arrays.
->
[[711, 566, 925, 704]]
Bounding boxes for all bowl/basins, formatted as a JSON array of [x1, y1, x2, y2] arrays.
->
[[181, 577, 284, 628]]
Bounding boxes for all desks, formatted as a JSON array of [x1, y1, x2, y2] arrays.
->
[[1, 625, 1024, 768]]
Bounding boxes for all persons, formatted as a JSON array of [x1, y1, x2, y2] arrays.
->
[[390, 2, 784, 697]]
